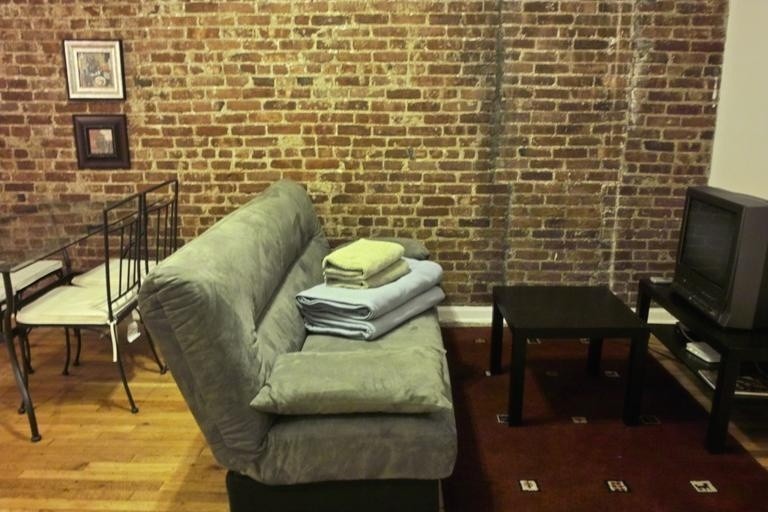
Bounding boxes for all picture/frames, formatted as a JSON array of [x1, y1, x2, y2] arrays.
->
[[63, 39, 126, 101], [73, 113, 131, 170]]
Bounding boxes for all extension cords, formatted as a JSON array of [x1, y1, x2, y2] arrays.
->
[[650, 276, 673, 284]]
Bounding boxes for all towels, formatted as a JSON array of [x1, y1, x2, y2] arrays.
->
[[322, 238, 405, 280], [324, 259, 409, 289]]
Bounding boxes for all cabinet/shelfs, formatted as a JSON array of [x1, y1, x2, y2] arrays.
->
[[631, 278, 768, 454]]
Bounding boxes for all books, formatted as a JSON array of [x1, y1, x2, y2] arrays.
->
[[685, 338, 729, 365], [697, 367, 768, 398]]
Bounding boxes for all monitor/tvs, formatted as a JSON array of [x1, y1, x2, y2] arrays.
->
[[672, 186, 768, 329]]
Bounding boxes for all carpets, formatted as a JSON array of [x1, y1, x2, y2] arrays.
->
[[440, 323, 768, 512]]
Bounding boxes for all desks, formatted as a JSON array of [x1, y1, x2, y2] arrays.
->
[[0, 196, 167, 441]]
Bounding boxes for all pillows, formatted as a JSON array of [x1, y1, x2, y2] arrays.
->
[[249, 347, 449, 416], [334, 237, 430, 264]]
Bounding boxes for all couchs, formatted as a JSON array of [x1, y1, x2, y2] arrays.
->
[[136, 177, 459, 512]]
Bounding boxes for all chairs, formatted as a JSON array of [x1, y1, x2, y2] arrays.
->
[[71, 179, 179, 376], [4, 259, 65, 373], [16, 192, 166, 414]]
